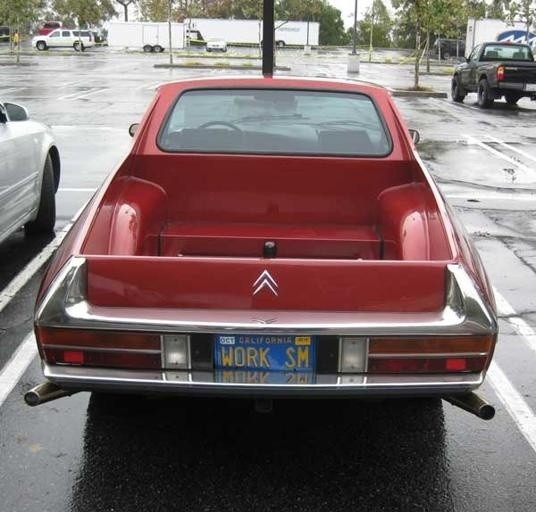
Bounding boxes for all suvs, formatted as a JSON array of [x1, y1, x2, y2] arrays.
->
[[32, 29, 95, 52], [435, 39, 465, 59]]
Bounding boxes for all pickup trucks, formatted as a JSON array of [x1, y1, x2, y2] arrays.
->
[[23, 76, 499, 423], [452, 42, 536, 108]]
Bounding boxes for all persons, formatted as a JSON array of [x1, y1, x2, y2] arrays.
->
[[12, 28, 21, 52]]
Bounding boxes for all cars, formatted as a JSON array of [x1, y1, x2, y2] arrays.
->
[[1, 98, 61, 245], [207, 38, 227, 52]]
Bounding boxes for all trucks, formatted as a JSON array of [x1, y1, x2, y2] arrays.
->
[[466, 16, 536, 63]]
